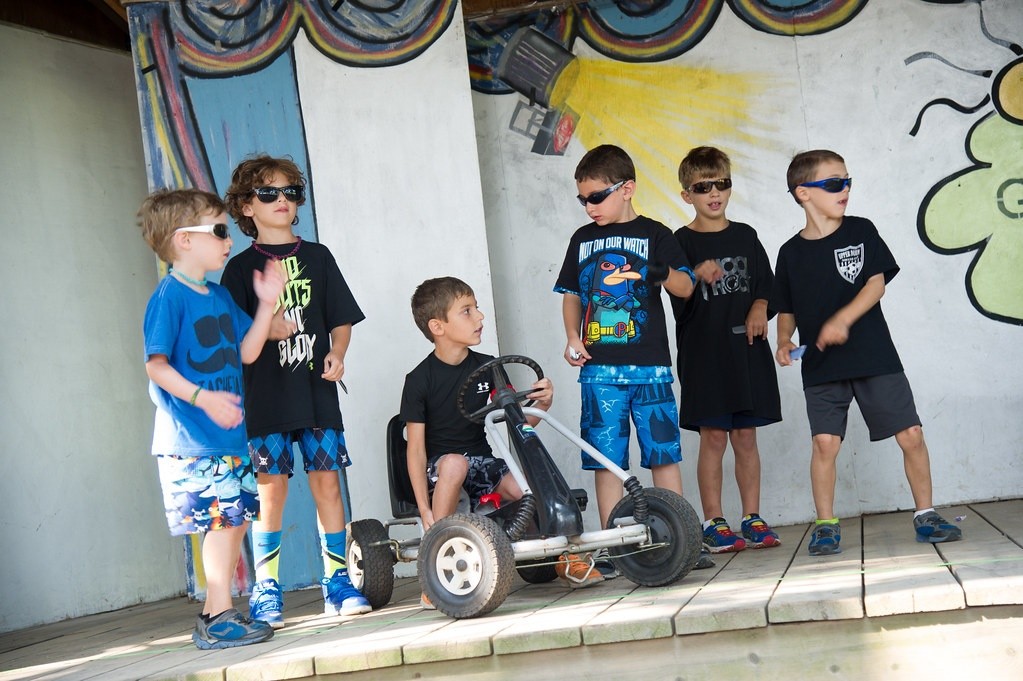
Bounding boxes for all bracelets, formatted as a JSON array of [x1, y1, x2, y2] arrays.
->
[[191, 387, 202, 406]]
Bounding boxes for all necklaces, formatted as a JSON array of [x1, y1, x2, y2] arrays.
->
[[252, 236, 302, 259], [169, 268, 207, 286]]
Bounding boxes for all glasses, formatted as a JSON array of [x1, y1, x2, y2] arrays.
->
[[687, 178, 732, 194], [173, 224, 231, 239], [789, 178, 851, 194], [577, 178, 632, 206], [253, 185, 304, 203]]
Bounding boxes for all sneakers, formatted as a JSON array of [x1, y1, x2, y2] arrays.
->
[[193, 608, 273, 650], [320, 572, 373, 618], [555, 555, 605, 590], [913, 512, 963, 545], [588, 550, 618, 581], [420, 590, 438, 611], [741, 513, 780, 549], [247, 580, 284, 629], [700, 518, 748, 556], [808, 518, 842, 555]]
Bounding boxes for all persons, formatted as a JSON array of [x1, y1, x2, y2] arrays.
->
[[665, 147, 784, 554], [137, 188, 287, 650], [399, 277, 604, 609], [224, 157, 372, 628], [551, 144, 716, 579], [774, 149, 963, 556]]
